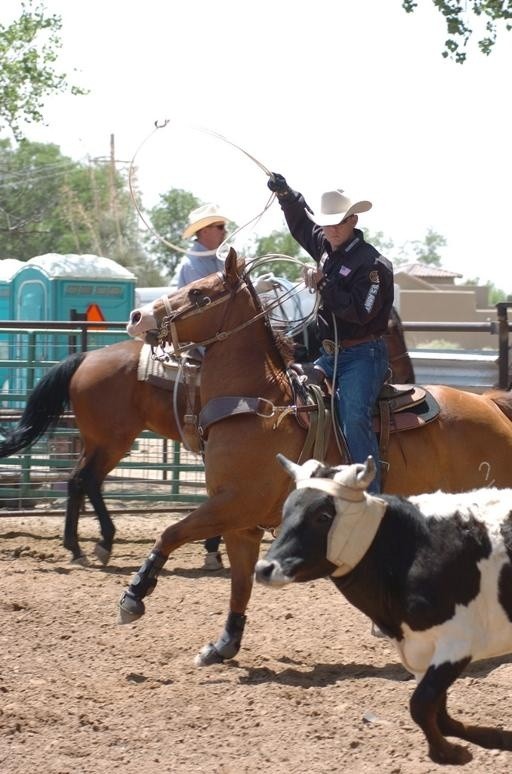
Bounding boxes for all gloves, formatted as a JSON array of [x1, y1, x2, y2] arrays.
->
[[251, 272, 279, 294], [263, 171, 295, 203]]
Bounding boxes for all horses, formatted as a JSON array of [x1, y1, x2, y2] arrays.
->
[[116, 246, 512, 667], [0, 303, 416, 571]]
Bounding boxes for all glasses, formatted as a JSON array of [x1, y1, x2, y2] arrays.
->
[[205, 223, 228, 230]]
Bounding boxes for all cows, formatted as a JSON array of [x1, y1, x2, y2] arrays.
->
[[253, 452, 512, 765]]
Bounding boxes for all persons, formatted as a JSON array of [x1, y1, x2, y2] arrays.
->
[[266, 170, 395, 496], [175, 202, 231, 290]]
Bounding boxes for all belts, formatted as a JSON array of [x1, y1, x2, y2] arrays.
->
[[321, 334, 380, 350]]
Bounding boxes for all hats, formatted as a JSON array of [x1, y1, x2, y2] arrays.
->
[[303, 187, 373, 230], [181, 201, 232, 238]]
